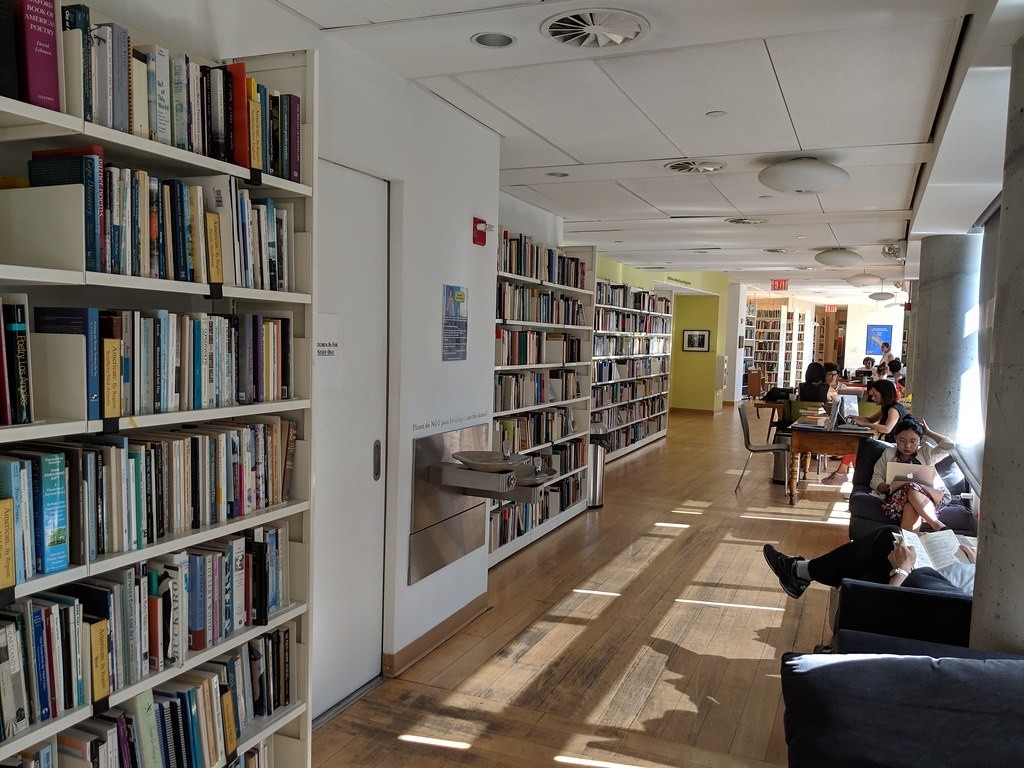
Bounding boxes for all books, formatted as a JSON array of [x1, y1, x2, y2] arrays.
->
[[742, 304, 825, 396], [892, 528, 971, 571], [791, 406, 828, 430], [0, 0, 306, 768], [488, 225, 673, 553]]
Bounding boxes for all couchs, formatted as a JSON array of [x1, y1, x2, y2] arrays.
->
[[780, 436, 1024, 768]]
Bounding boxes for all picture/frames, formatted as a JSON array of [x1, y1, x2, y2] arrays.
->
[[739, 336, 744, 348], [682, 330, 711, 352], [903, 329, 909, 343], [902, 343, 908, 357]]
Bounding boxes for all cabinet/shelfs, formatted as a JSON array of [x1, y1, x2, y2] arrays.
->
[[0, 47, 321, 768], [473, 244, 673, 570], [742, 302, 830, 400]]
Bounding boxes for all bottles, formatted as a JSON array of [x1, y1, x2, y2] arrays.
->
[[844, 369, 847, 379]]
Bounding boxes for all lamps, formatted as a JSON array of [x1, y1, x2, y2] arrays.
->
[[815, 248, 864, 268], [847, 269, 881, 288], [885, 292, 904, 308], [757, 157, 850, 196], [868, 278, 896, 301]]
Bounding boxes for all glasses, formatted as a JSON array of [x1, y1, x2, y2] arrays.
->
[[827, 373, 838, 378], [896, 438, 918, 445]]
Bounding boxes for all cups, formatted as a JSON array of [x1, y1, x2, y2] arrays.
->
[[503, 440, 511, 459], [534, 457, 543, 479], [863, 376, 867, 384], [789, 394, 796, 400]]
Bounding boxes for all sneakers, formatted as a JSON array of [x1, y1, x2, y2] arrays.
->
[[762, 543, 811, 599], [814, 644, 839, 654], [821, 470, 849, 485]]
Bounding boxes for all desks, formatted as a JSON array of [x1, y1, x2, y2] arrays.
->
[[753, 402, 788, 443], [787, 415, 875, 507]]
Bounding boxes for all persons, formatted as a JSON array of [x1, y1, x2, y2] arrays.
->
[[796, 362, 843, 460], [762, 525, 977, 653], [821, 342, 956, 532]]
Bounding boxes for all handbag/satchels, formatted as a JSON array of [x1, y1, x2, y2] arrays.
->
[[759, 387, 796, 402]]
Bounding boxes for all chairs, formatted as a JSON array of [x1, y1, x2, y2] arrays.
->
[[858, 401, 882, 418], [734, 403, 790, 496], [790, 401, 827, 475]]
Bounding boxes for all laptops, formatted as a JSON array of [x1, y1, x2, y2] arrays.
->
[[886, 461, 934, 489], [855, 370, 872, 377], [821, 405, 849, 424]]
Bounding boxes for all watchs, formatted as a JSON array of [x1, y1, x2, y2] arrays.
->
[[889, 568, 908, 578]]
